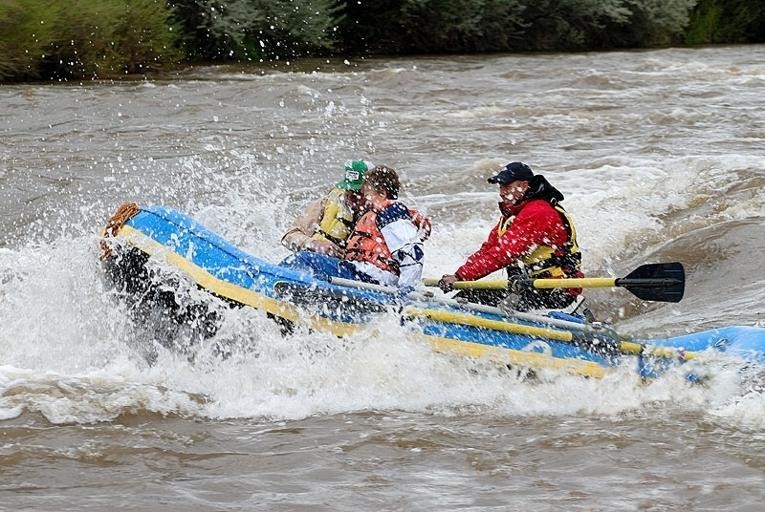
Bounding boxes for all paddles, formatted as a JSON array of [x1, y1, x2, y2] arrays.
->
[[420, 263, 685, 304]]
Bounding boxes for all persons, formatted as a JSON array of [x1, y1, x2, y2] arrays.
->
[[281, 161, 376, 258], [279, 163, 424, 289], [440, 162, 584, 313]]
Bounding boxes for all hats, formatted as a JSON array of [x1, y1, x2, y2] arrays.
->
[[336, 160, 375, 192], [487, 161, 535, 185], [362, 165, 401, 192]]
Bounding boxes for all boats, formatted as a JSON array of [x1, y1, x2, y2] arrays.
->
[[97, 206, 764, 385], [101, 201, 764, 388]]
